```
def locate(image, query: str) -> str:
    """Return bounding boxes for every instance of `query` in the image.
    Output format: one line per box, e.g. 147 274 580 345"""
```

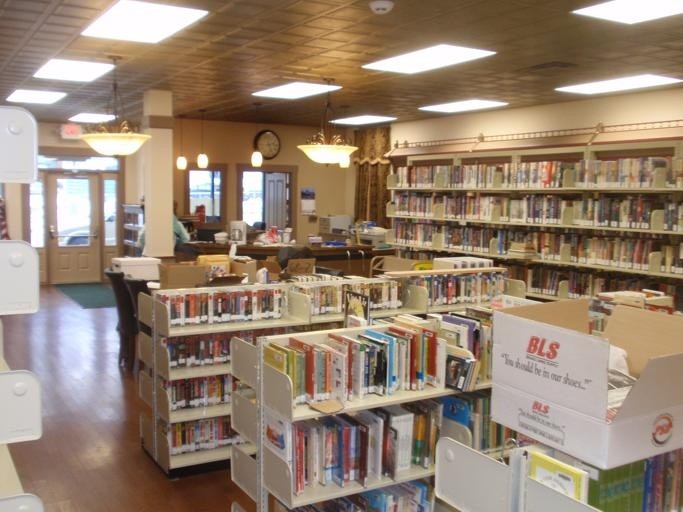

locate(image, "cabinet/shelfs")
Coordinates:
319 214 351 242
432 426 683 512
0 104 45 511
230 304 498 512
120 203 145 257
387 147 683 314
137 266 526 476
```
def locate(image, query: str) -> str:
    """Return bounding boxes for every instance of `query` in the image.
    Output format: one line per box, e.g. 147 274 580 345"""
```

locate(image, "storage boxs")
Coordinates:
490 298 683 470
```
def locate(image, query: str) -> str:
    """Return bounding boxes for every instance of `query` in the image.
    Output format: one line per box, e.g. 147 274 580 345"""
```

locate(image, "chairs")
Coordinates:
102 267 148 371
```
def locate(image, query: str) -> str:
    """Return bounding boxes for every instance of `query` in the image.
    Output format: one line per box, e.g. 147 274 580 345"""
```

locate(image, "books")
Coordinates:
160 415 250 458
291 388 517 499
396 245 441 261
395 190 500 221
393 217 683 276
405 271 507 309
262 304 494 406
395 156 499 189
494 258 682 337
163 322 343 371
288 272 407 316
501 154 683 191
159 372 242 413
502 192 683 231
525 447 683 512
156 282 290 327
291 478 437 512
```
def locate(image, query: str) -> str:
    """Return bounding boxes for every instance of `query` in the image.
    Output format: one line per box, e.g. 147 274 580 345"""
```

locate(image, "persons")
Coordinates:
137 213 190 256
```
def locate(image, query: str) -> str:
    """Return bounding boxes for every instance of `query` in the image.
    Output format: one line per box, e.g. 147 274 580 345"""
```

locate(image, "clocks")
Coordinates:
253 130 281 160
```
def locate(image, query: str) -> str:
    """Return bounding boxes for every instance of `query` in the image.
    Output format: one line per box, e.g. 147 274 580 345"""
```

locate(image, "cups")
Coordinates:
283 233 290 244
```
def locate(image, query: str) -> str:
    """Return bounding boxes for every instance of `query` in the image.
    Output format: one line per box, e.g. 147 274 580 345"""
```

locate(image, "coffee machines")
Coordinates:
229 221 246 245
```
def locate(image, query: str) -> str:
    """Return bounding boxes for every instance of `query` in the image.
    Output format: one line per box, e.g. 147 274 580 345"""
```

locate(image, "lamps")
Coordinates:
296 78 358 169
250 103 264 169
79 54 152 156
176 114 187 170
196 109 209 169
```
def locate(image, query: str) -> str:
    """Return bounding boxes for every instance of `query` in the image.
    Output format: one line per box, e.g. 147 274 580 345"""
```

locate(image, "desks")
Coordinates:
188 241 373 259
